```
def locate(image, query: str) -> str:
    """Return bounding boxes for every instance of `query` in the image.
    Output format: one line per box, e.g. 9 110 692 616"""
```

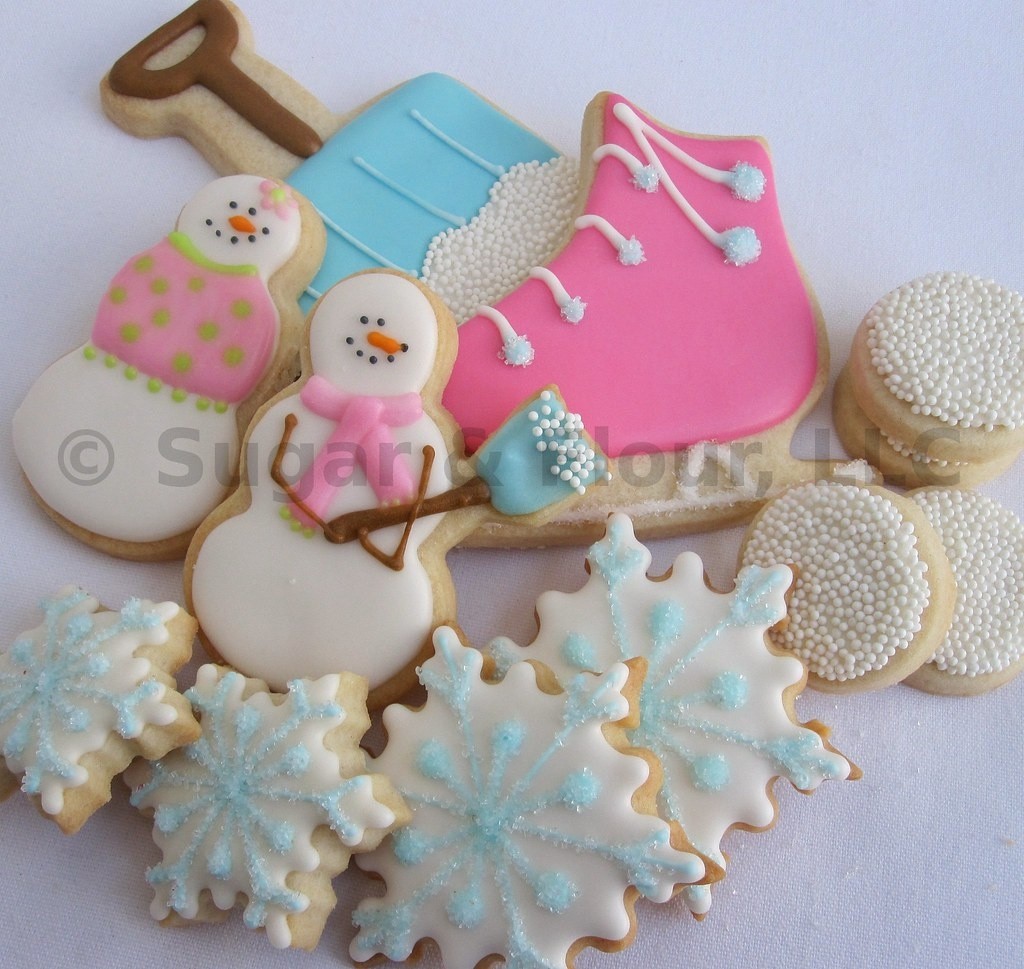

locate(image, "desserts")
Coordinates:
2 93 1022 969
